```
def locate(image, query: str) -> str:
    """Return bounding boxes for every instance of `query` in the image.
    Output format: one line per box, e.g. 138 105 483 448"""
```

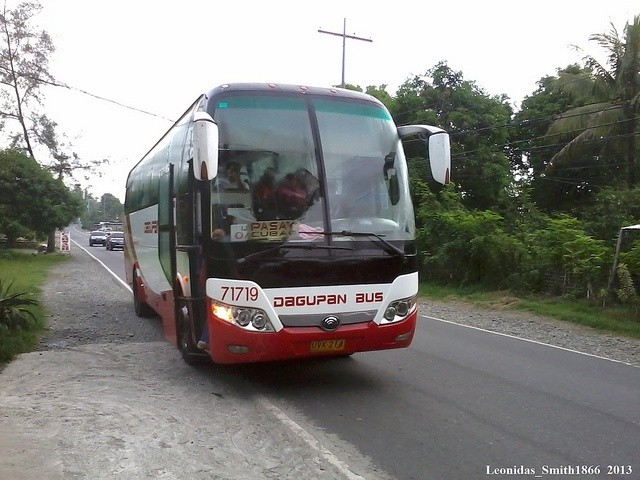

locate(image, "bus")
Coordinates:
123 81 451 365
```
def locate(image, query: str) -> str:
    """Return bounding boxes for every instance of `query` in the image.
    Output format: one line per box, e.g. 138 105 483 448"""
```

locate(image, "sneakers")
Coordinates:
198 341 209 350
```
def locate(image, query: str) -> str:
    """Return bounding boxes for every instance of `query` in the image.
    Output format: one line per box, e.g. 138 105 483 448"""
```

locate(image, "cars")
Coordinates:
106 232 124 251
89 231 107 246
96 228 112 232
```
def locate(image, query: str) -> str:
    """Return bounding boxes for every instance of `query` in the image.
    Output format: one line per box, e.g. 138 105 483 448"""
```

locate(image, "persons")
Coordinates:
214 160 247 193
343 185 378 219
213 214 243 242
